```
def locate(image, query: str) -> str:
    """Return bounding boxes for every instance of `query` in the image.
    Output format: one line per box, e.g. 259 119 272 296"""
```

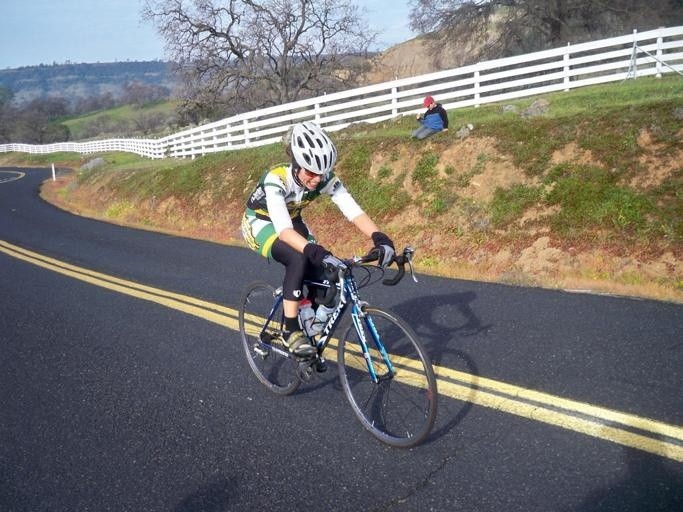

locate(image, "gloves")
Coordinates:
370 230 395 271
304 243 340 282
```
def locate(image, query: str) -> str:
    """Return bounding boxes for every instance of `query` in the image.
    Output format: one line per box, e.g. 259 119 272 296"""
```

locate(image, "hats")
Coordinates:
424 96 434 107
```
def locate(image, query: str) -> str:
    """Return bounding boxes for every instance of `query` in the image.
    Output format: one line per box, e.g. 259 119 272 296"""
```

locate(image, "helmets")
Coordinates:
289 121 338 174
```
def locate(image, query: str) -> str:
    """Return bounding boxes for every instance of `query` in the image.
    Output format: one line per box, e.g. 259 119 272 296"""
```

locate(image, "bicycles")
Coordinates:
235 246 439 450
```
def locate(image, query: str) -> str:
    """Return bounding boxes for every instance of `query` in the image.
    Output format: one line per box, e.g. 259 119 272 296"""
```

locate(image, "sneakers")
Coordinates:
314 358 327 372
280 330 317 359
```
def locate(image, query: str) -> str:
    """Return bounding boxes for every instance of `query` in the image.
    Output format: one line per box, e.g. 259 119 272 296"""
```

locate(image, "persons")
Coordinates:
238 122 396 374
408 96 449 143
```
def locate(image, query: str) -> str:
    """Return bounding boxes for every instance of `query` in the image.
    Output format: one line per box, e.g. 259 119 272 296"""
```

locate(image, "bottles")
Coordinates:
310 298 336 333
297 296 318 338
